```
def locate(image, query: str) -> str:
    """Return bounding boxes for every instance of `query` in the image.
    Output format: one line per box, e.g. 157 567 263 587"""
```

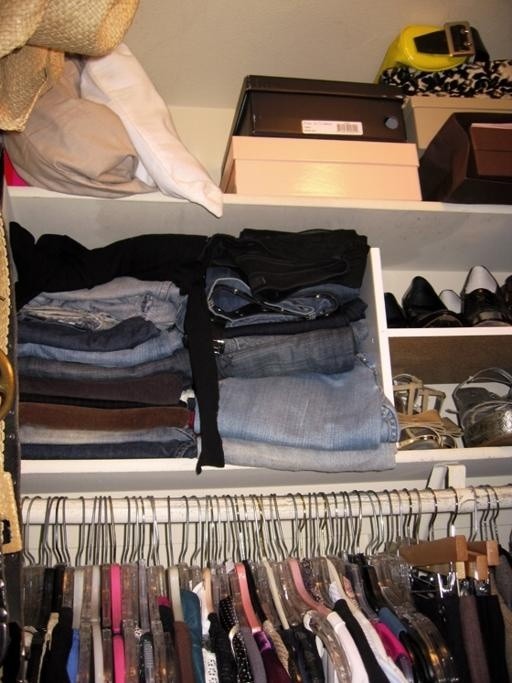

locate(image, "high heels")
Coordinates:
445 366 512 447
384 265 512 328
393 374 464 449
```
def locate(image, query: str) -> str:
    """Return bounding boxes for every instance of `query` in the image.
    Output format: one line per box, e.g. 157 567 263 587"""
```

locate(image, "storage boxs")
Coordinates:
218 75 512 205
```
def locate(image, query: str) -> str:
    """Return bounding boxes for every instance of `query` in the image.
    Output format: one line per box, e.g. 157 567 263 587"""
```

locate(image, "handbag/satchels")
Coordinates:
374 20 474 86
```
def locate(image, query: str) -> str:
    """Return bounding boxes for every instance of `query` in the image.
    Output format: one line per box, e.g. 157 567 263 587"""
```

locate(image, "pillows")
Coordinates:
78 37 225 221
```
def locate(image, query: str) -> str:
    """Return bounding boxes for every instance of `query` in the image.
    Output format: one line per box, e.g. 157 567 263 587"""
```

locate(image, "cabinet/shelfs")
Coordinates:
2 184 512 474
